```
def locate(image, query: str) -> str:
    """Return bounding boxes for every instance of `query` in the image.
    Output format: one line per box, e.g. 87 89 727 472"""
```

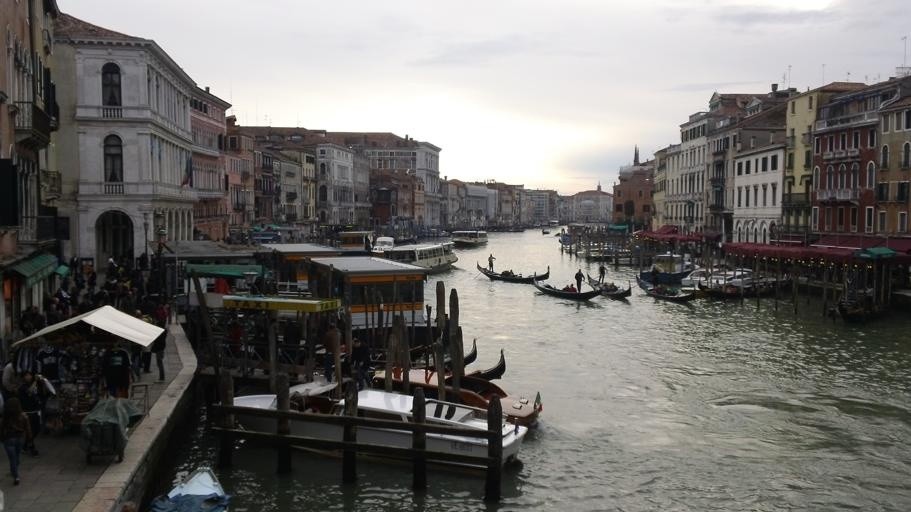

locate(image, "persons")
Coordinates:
598 263 608 283
1 221 445 486
864 283 874 313
488 254 497 273
568 284 576 291
651 266 660 292
574 269 585 293
563 285 569 291
713 279 723 289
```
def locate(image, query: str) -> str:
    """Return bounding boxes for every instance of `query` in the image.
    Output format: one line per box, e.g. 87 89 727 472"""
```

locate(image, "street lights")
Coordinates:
140 211 150 272
158 224 169 325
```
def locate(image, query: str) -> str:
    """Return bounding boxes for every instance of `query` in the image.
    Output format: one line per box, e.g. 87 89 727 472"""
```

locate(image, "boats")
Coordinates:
527 241 791 304
148 463 228 512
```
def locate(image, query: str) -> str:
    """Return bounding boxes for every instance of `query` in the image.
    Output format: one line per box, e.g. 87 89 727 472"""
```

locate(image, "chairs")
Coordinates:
25 335 151 464
